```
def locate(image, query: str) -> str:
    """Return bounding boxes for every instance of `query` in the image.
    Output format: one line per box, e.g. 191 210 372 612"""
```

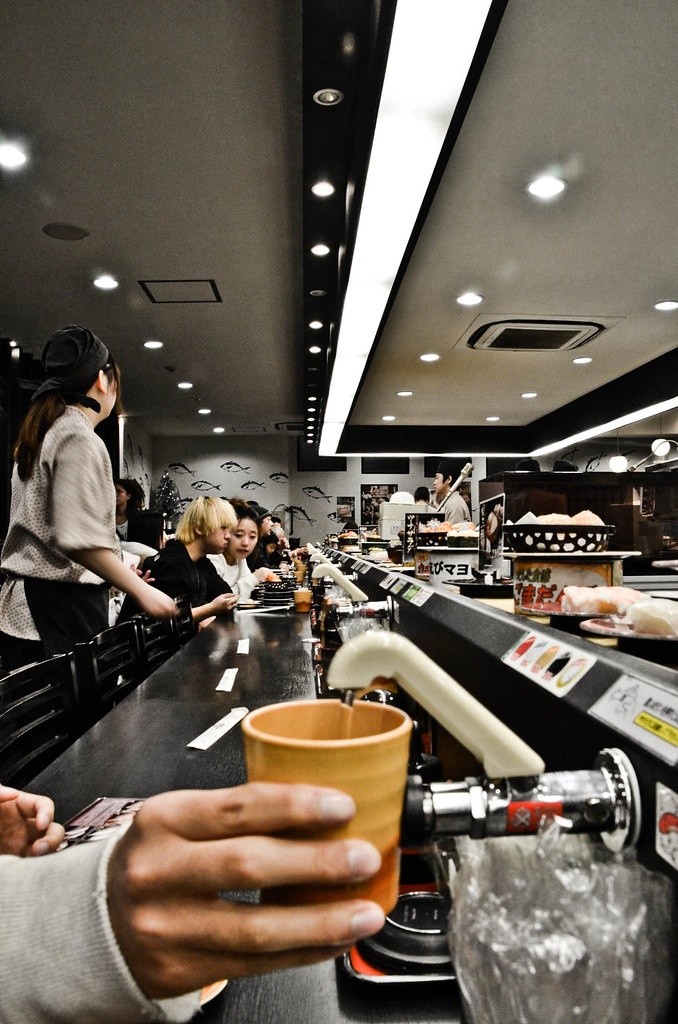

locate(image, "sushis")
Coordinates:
559 584 678 636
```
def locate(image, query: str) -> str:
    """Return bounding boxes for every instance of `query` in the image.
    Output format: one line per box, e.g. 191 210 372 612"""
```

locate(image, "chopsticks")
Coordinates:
225 594 240 604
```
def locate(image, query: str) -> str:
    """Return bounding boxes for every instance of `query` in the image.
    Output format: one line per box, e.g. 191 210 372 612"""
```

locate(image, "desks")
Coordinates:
21 607 316 825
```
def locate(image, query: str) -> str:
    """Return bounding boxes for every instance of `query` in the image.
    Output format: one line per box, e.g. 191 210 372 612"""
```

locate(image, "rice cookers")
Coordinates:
513 557 624 624
415 546 430 580
429 547 479 591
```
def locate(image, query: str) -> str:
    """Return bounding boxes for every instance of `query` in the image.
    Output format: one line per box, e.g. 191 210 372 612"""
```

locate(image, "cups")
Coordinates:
293 571 304 582
294 560 306 576
294 590 312 612
241 697 414 915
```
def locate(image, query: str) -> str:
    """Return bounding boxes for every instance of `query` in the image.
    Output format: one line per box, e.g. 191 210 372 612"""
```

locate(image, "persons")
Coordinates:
397 460 472 540
116 496 309 632
116 477 160 549
0 781 386 1024
0 325 181 665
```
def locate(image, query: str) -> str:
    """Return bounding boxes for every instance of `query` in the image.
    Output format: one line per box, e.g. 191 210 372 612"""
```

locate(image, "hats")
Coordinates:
251 505 272 520
437 460 462 479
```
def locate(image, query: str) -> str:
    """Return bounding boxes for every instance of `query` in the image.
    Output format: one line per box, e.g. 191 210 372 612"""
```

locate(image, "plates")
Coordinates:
238 603 262 608
520 602 607 637
580 617 678 662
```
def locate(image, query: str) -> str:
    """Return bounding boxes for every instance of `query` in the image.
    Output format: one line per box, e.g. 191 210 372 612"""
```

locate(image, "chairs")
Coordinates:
0 595 196 791
